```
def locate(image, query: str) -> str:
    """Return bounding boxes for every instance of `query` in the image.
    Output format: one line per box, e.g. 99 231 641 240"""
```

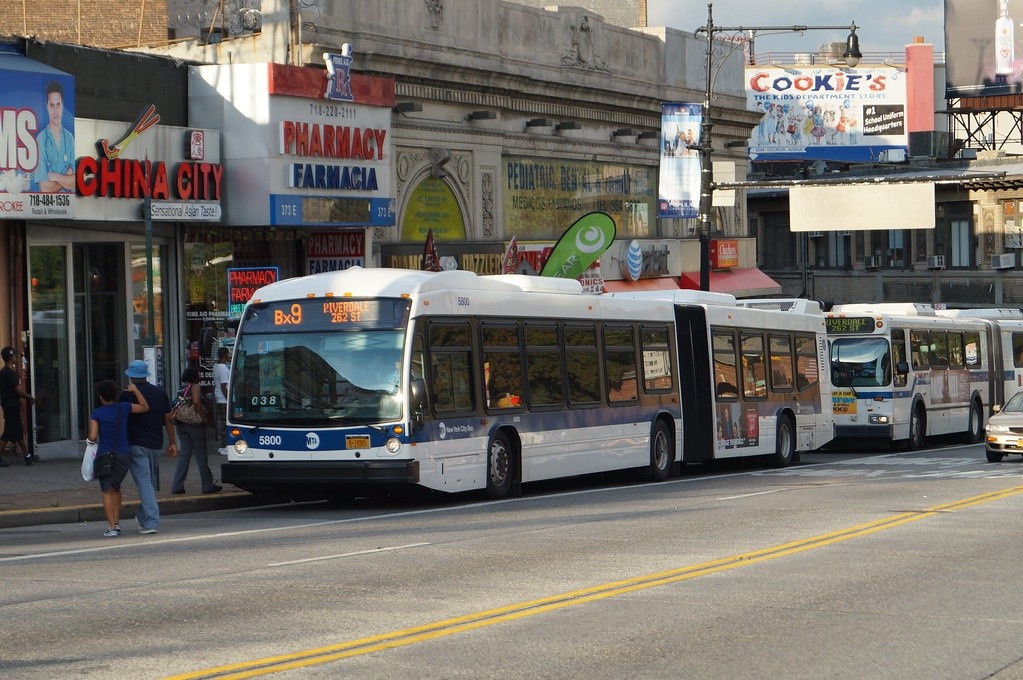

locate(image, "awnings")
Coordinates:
682 267 782 298
602 278 680 293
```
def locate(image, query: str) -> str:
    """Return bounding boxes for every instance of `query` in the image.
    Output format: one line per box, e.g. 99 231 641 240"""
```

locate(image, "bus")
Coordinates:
221 264 835 516
131 255 163 345
826 302 1023 450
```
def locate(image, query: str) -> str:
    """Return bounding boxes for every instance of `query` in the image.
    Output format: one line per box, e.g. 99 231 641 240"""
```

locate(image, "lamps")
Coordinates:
467 110 496 122
526 118 552 128
613 128 639 137
556 121 582 131
638 131 662 138
724 140 749 148
394 102 423 115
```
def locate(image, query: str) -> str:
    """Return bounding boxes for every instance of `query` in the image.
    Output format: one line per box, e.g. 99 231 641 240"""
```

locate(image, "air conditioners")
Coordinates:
808 231 824 237
837 231 852 236
926 255 945 267
991 253 1015 269
864 256 882 267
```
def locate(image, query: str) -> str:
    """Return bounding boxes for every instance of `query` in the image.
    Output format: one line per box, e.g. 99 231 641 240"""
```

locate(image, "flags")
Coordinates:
503 238 520 274
421 228 440 271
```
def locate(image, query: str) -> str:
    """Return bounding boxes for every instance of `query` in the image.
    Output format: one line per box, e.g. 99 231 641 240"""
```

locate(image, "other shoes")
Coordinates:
138 527 158 534
217 448 227 455
25 454 39 464
171 489 186 494
201 484 222 493
0 458 9 467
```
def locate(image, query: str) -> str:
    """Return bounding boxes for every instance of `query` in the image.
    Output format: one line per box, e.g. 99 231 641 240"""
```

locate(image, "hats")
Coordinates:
124 360 150 378
1 347 18 361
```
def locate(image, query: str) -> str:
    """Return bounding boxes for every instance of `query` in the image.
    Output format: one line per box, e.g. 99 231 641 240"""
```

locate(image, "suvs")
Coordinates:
33 309 67 338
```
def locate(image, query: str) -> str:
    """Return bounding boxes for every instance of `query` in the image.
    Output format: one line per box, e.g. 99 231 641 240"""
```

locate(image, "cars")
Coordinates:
983 386 1023 462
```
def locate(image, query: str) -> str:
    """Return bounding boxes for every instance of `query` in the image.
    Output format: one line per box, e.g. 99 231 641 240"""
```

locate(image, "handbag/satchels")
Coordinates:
92 452 114 480
170 384 202 425
80 439 98 481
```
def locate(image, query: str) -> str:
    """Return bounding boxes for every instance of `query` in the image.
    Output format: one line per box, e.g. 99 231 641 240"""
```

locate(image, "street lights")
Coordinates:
697 23 863 289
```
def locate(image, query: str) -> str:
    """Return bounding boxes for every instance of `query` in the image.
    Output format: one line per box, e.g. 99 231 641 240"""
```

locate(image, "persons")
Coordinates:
171 368 223 494
841 363 871 376
212 347 230 455
88 380 149 536
717 407 748 450
664 125 698 156
118 360 177 534
0 346 39 467
34 80 75 193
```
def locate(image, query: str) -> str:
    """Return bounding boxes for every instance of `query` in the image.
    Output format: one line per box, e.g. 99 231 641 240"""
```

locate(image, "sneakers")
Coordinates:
103 530 117 537
108 524 120 533
